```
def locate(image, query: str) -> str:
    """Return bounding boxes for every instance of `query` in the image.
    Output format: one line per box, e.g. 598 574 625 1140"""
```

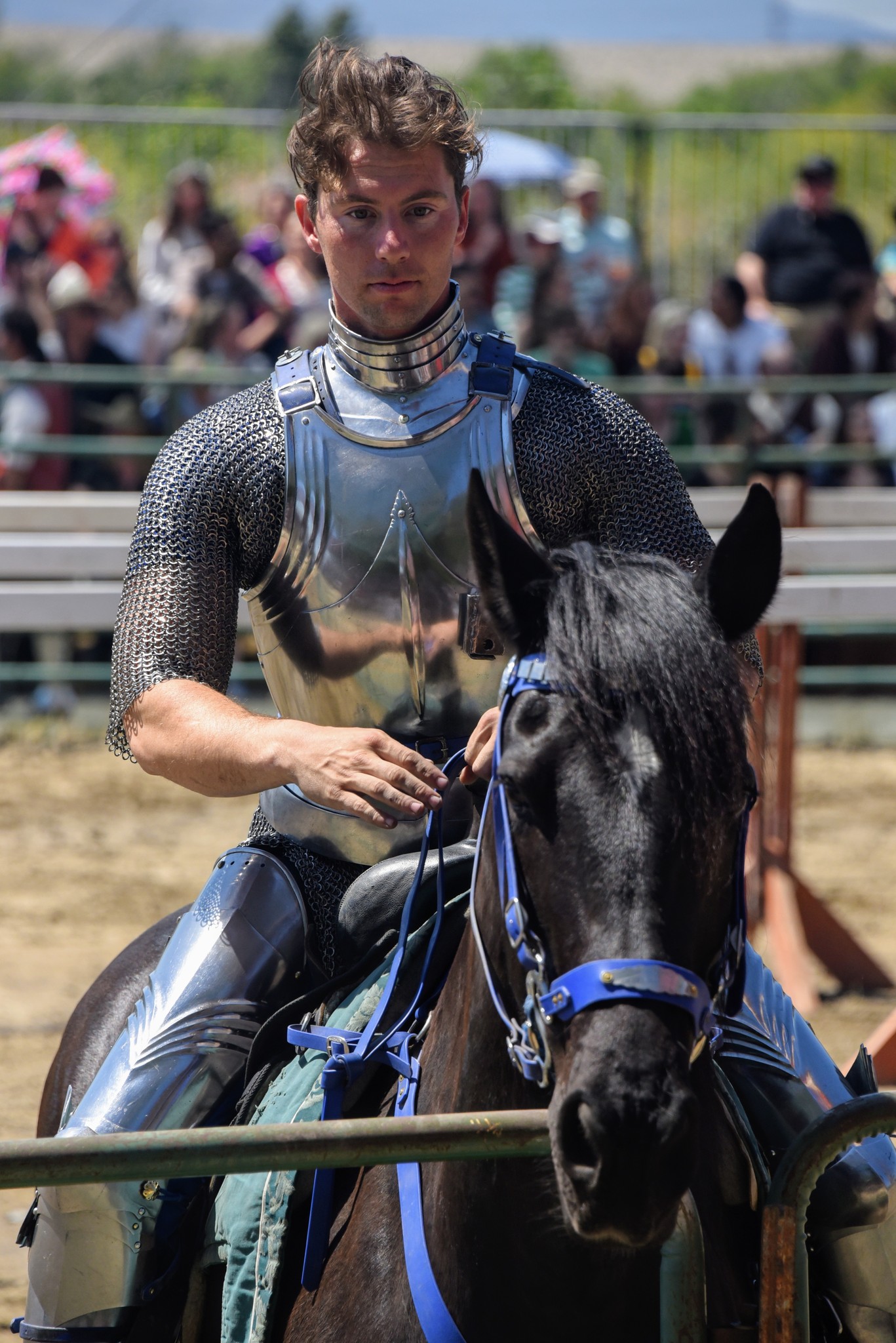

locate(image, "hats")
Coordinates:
560 156 603 199
797 158 836 185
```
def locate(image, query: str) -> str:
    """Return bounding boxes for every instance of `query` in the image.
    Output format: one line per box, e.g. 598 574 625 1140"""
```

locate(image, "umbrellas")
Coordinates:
465 129 578 182
0 123 115 219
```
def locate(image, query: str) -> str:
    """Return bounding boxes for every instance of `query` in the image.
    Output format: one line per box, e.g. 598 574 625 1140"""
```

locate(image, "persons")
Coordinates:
12 35 896 1343
0 155 896 528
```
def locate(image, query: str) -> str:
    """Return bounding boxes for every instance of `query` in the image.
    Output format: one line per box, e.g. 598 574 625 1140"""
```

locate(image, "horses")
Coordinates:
33 466 784 1343
